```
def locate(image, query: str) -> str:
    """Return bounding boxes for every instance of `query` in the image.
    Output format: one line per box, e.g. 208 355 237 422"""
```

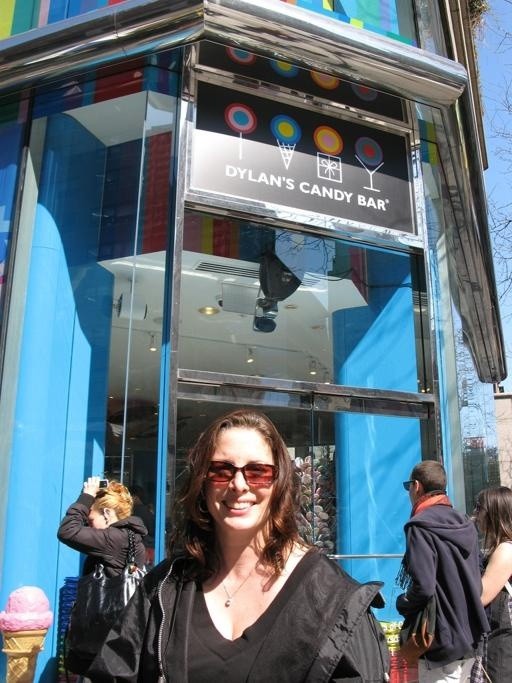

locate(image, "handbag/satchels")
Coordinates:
71 565 145 654
399 594 436 664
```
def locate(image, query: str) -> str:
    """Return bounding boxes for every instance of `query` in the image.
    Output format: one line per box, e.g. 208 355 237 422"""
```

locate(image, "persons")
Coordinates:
393 460 490 682
78 406 393 683
470 489 510 682
58 476 149 682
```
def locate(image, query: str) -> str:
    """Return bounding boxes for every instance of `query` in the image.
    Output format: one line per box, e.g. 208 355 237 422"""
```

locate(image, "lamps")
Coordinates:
246 345 256 363
148 331 158 352
309 356 317 375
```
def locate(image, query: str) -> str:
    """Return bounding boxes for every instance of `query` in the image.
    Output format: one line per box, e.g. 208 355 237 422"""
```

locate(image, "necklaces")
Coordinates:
217 561 263 606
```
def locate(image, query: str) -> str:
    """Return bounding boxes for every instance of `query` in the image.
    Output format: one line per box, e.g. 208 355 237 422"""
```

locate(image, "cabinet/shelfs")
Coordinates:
105 451 135 492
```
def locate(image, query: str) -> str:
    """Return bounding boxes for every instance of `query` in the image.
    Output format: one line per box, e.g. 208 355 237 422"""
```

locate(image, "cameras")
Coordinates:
100 480 108 489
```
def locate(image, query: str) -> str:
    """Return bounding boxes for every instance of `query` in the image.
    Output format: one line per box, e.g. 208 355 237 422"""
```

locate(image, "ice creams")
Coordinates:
0 586 53 683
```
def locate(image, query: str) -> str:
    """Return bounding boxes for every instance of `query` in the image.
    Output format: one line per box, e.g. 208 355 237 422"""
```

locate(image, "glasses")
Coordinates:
207 461 277 483
403 480 414 490
